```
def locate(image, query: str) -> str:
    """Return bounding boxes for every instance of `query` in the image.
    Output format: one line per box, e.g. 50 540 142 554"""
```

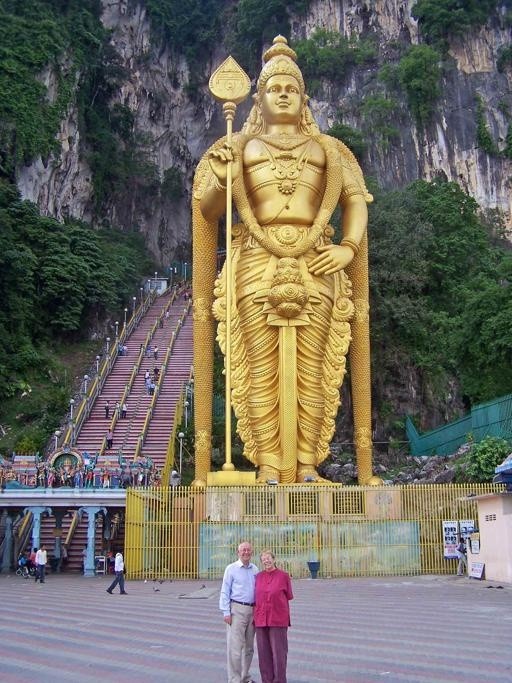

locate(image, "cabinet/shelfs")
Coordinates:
95 556 107 576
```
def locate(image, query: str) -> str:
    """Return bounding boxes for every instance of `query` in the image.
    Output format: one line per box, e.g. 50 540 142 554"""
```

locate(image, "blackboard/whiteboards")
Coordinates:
469 562 484 579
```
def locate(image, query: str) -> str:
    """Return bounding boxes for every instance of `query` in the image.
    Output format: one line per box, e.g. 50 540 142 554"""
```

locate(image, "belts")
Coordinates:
229 597 256 608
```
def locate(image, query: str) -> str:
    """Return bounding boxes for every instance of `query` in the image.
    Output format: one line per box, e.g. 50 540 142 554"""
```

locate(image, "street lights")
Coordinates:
189 378 194 413
176 430 185 475
115 262 188 337
105 336 111 353
84 373 89 394
54 430 61 450
184 400 190 429
96 355 101 373
69 397 75 420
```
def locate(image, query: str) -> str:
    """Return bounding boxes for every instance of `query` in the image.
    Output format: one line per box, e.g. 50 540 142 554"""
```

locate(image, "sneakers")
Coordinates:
106 590 113 594
120 591 127 594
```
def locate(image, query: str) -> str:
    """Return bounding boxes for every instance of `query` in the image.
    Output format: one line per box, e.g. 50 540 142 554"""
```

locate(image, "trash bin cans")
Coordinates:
48 558 60 572
307 562 320 579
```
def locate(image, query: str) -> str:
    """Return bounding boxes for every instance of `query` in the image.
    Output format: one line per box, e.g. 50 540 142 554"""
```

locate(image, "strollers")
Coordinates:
16 554 36 576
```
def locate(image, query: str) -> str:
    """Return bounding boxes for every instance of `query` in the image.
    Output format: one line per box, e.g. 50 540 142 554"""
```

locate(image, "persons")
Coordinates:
254 549 293 683
456 537 468 576
219 542 260 683
200 34 367 487
